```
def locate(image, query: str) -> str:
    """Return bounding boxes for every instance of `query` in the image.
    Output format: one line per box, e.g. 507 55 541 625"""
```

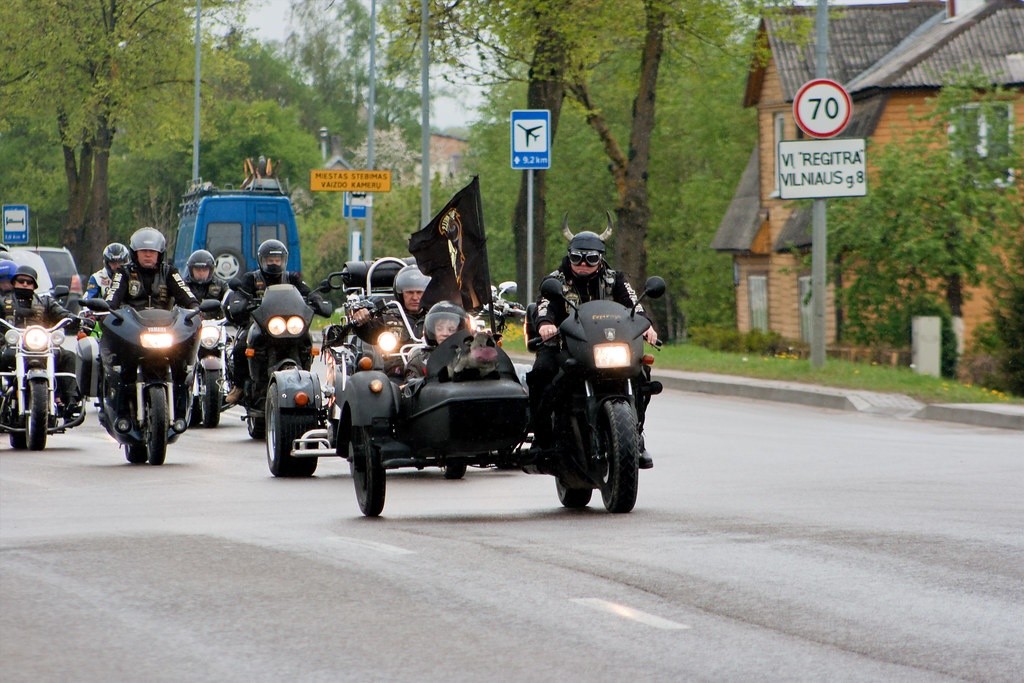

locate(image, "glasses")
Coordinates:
569 248 601 266
15 277 34 284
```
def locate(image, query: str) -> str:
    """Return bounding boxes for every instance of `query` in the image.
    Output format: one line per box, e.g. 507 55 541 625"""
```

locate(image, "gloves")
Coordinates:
66 313 80 330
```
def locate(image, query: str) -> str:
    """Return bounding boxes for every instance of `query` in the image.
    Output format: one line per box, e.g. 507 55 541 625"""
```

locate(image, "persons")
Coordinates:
0 227 474 420
521 230 657 469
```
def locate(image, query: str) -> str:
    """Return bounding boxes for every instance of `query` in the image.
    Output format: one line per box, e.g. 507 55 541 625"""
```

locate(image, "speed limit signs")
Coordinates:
791 78 855 139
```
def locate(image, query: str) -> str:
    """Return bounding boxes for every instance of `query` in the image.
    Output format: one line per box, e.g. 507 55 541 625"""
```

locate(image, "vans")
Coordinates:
172 178 302 282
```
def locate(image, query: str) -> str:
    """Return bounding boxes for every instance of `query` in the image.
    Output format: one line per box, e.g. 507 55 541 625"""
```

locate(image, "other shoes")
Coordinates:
225 385 244 403
57 403 84 424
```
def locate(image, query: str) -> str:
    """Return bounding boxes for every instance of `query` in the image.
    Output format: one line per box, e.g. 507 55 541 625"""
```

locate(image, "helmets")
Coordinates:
102 243 130 279
567 230 606 283
10 265 38 289
392 265 432 304
423 300 468 345
257 239 289 279
129 227 166 275
0 260 18 282
0 250 13 261
187 249 217 288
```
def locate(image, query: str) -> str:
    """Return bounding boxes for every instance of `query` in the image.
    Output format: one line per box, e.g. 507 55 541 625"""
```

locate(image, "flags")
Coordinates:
407 175 492 319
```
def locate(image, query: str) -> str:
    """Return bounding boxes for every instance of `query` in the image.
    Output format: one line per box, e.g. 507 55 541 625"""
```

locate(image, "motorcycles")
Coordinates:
0 311 86 450
86 297 220 465
264 257 669 517
227 272 346 439
65 299 240 428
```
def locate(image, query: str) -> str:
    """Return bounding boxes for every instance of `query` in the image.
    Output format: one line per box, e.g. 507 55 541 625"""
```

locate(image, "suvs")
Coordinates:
13 246 84 311
